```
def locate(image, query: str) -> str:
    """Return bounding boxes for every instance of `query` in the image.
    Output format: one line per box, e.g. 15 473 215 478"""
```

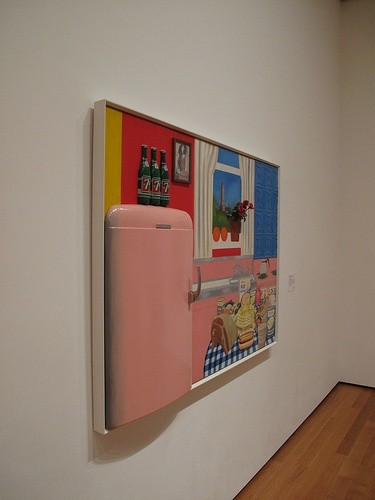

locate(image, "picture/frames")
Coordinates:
91 98 282 434
